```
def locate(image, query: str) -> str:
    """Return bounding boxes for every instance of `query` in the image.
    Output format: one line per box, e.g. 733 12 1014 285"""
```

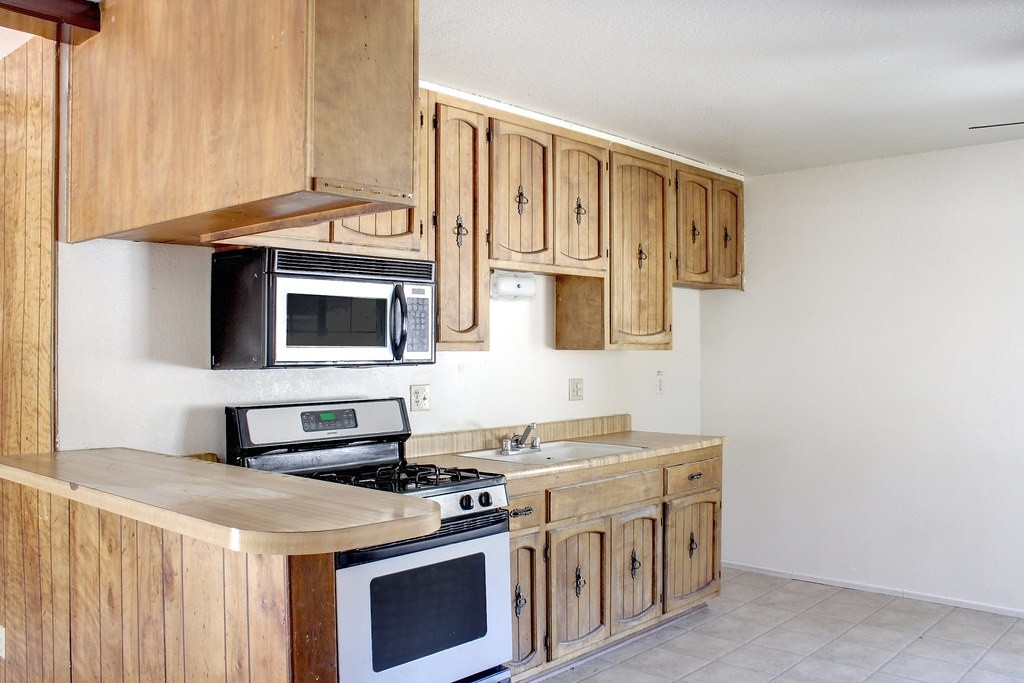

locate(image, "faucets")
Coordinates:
500 422 542 455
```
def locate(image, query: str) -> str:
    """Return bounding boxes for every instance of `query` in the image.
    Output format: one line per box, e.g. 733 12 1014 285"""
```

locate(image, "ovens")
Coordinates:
334 510 512 683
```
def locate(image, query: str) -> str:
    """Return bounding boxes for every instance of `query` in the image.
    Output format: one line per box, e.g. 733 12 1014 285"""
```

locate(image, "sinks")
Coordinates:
458 438 646 465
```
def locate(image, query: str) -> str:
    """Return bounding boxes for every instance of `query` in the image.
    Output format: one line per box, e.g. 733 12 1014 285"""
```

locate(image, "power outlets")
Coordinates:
569 377 583 401
410 383 431 411
657 370 664 395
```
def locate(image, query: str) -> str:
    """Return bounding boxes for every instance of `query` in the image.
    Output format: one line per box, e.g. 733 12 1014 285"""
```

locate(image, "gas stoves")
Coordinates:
224 398 510 520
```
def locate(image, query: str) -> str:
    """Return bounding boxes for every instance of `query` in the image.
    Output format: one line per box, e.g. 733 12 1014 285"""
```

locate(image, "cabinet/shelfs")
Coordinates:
503 474 550 683
66 0 422 246
546 455 666 666
660 444 723 619
210 78 425 261
489 106 611 278
425 88 489 351
670 158 744 292
553 124 675 351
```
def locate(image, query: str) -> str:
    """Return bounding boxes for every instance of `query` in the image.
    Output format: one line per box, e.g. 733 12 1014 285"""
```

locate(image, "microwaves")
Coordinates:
210 247 438 370
